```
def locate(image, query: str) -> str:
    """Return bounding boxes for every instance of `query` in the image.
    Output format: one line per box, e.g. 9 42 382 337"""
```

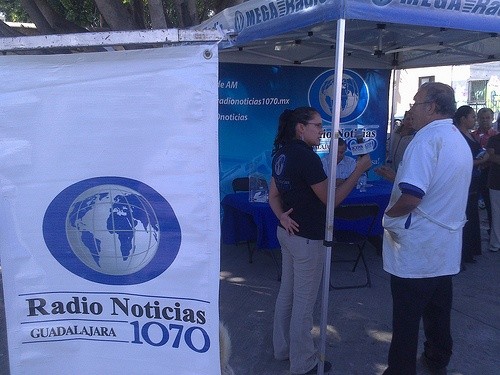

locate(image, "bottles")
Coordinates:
356 173 367 192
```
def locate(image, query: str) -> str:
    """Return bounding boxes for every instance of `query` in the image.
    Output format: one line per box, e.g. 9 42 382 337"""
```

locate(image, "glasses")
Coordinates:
301 121 323 128
409 102 435 110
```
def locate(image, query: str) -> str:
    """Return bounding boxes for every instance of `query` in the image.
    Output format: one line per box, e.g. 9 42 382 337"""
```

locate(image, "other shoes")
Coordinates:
489 245 499 252
465 254 477 263
304 360 332 375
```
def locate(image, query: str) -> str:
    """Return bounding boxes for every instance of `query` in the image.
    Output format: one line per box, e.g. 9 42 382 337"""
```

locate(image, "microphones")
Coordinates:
351 129 374 157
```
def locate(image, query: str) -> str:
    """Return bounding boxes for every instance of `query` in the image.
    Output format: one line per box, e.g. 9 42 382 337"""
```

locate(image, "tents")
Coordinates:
191 0 500 375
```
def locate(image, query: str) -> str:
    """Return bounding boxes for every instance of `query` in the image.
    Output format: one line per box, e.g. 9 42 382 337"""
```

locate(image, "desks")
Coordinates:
221 180 393 282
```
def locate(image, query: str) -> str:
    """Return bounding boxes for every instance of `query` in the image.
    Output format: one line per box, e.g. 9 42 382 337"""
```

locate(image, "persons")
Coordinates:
321 137 357 187
392 111 416 172
454 106 500 270
374 82 473 375
269 107 372 375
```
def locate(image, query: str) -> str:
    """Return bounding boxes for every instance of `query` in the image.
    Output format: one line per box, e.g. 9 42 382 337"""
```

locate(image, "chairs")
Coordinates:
329 203 379 289
232 177 269 264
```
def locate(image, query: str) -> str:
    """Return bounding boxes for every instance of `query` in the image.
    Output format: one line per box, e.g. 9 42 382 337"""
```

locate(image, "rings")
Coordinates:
368 163 371 166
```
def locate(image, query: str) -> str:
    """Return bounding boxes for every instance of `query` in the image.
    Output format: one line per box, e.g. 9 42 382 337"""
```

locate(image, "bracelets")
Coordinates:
354 172 360 178
